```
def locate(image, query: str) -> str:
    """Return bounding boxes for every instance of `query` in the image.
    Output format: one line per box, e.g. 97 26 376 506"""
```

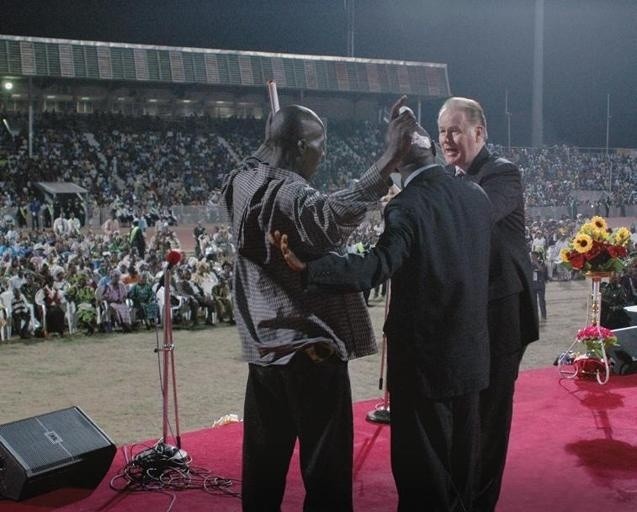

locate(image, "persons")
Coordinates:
216 94 415 511
2 94 636 340
265 120 493 511
436 96 539 512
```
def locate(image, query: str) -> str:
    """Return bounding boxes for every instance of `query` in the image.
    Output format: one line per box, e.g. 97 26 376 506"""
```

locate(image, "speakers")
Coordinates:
0 406 118 502
624 305 637 327
602 327 637 377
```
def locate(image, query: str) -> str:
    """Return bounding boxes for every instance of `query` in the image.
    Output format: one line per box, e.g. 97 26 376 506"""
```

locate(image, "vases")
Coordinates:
574 357 612 379
588 277 603 328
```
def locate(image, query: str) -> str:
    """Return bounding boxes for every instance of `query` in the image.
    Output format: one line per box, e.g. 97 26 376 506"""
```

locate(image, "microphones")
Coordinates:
265 78 282 115
157 244 183 294
390 183 401 195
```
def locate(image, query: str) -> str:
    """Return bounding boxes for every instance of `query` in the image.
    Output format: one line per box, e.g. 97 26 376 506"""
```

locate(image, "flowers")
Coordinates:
577 321 621 360
560 217 633 281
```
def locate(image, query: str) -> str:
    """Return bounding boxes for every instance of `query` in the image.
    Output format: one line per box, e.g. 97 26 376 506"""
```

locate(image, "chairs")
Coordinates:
0 249 234 342
